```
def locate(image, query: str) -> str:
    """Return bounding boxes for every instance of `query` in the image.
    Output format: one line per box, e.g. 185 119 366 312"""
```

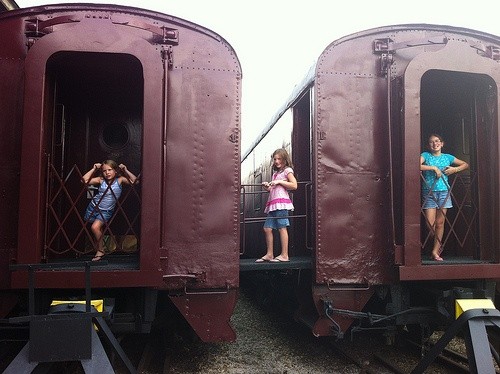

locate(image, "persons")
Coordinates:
254 148 298 262
80 160 138 263
419 133 469 260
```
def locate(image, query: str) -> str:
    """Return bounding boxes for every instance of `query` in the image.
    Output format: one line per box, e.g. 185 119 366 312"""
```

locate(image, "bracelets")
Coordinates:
454 167 461 173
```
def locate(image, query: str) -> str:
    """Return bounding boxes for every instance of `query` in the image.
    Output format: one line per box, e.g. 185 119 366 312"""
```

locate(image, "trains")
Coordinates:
0 2 243 374
241 23 500 374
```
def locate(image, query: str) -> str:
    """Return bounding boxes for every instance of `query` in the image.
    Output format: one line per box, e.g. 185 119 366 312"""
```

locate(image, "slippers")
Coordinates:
270 257 290 263
429 254 443 261
255 258 274 263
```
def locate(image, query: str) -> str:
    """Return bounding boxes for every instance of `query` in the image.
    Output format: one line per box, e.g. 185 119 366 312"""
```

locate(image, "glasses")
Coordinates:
430 139 441 143
